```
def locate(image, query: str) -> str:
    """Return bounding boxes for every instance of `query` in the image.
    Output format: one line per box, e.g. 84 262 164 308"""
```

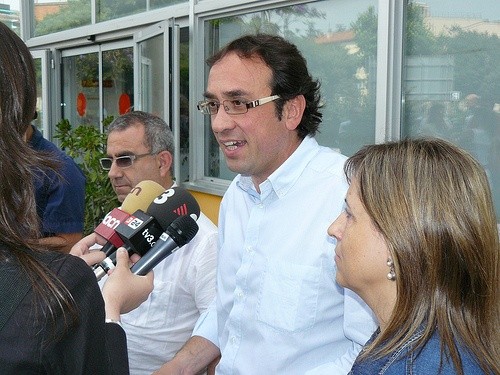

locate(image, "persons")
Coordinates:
21 114 86 250
196 33 381 375
0 18 155 375
327 137 500 375
80 111 221 375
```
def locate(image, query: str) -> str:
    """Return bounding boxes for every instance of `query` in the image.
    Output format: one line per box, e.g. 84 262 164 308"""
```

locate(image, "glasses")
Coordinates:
197 95 281 116
98 153 155 171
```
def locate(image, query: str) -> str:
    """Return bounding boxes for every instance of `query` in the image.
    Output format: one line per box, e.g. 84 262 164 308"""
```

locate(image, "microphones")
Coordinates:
129 215 198 275
93 186 200 282
89 180 166 271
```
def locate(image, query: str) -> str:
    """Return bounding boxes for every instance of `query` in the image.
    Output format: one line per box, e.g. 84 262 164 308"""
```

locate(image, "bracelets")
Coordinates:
105 318 123 329
